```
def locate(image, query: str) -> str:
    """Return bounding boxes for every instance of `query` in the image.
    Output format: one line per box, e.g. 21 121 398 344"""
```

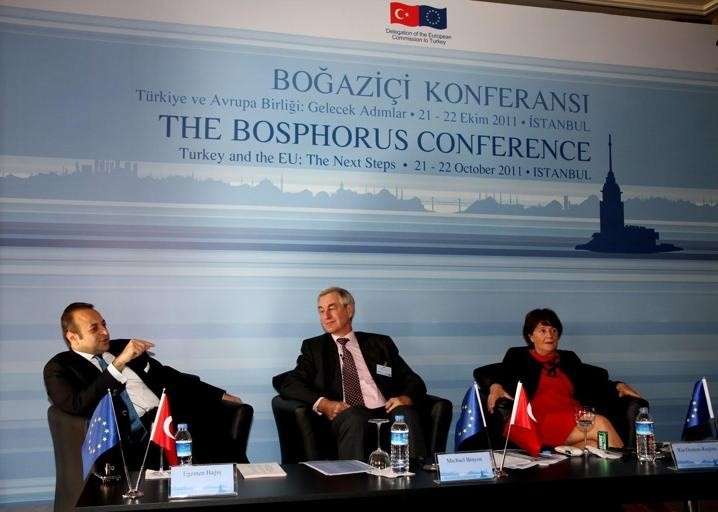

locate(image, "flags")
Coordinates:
150 393 179 466
81 394 119 482
501 381 542 458
681 377 716 441
454 383 487 451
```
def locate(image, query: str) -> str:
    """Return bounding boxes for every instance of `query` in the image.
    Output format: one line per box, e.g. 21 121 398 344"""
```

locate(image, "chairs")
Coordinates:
269 394 454 468
468 389 649 455
47 397 254 512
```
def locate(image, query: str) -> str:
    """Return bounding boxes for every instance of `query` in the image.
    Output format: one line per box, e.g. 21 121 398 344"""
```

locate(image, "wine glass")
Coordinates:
367 418 390 469
575 405 596 452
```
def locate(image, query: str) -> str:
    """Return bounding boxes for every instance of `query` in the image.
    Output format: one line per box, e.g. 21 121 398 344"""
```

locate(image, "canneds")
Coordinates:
597 431 607 450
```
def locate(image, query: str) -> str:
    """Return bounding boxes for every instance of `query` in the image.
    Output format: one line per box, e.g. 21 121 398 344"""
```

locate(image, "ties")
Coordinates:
337 336 365 409
92 353 148 443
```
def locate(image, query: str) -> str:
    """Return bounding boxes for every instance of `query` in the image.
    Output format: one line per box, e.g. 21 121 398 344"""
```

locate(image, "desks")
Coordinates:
82 440 718 512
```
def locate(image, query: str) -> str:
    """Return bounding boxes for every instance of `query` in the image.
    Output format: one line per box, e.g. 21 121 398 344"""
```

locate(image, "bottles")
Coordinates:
390 414 410 474
634 406 657 463
172 422 193 467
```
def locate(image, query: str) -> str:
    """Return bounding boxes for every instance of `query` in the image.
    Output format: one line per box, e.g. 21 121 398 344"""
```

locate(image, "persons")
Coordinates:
484 306 646 450
40 302 251 470
271 286 434 461
